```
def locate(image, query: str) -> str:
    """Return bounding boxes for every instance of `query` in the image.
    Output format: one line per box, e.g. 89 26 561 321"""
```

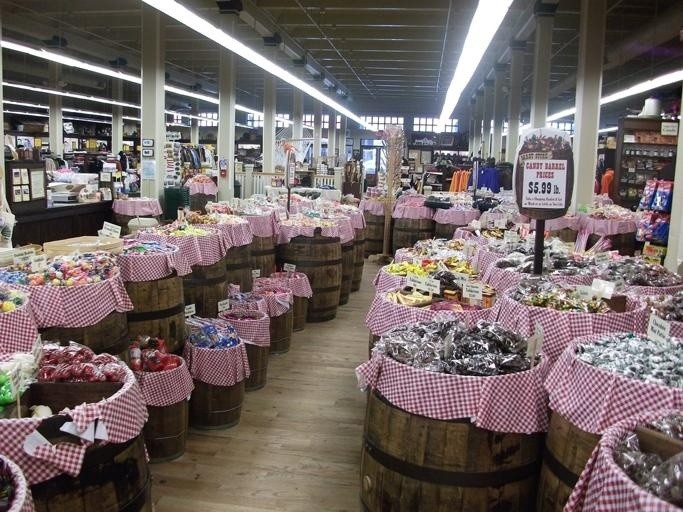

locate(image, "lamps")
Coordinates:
439 0 514 126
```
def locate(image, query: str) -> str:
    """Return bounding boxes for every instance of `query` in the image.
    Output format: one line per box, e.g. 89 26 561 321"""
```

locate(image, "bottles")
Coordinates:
16 145 42 161
625 145 673 158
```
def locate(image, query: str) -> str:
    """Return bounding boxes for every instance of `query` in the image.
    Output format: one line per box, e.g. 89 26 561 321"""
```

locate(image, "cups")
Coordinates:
18 125 24 133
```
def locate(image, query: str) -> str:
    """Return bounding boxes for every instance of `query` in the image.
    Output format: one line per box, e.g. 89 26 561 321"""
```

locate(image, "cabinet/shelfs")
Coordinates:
613 118 680 207
5 160 47 216
